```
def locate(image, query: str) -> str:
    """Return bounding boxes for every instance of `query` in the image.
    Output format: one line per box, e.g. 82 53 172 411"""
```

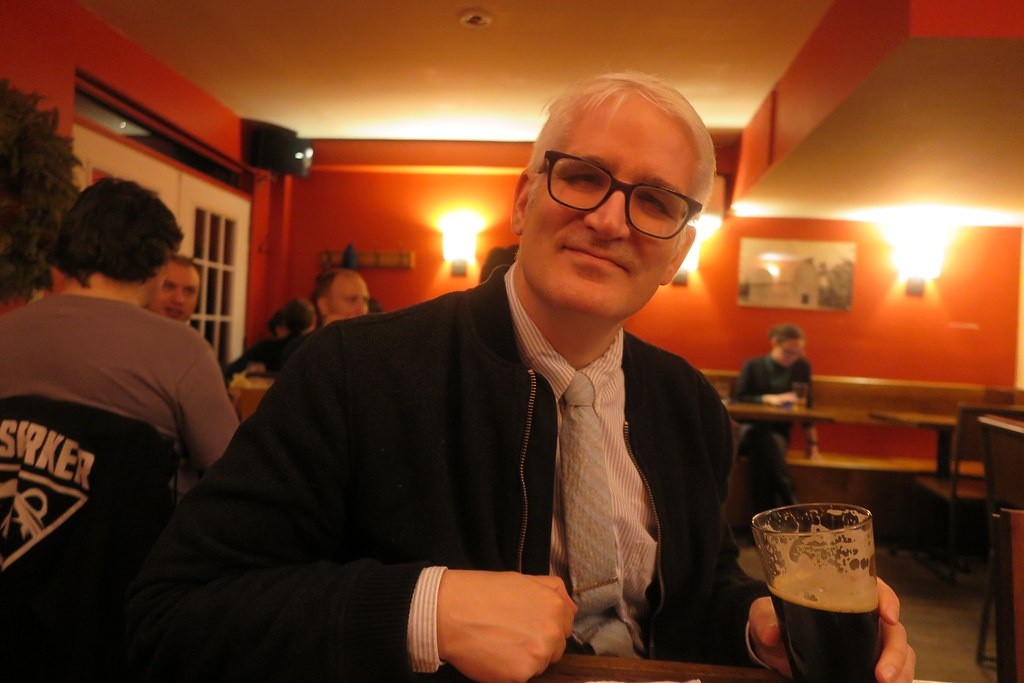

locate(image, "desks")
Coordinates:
722 402 843 424
868 409 974 576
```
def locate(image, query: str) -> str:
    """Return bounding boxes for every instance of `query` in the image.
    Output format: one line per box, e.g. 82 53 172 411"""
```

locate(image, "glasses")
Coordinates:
538 150 702 239
779 344 805 360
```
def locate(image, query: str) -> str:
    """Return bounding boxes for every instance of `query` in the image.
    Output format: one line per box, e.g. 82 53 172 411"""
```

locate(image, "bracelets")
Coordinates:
806 440 815 445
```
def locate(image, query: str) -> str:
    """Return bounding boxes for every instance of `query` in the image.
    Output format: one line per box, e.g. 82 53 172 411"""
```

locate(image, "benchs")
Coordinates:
698 372 984 547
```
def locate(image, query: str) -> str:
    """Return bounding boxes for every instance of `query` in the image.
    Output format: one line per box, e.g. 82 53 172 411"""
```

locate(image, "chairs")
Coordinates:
914 404 1024 683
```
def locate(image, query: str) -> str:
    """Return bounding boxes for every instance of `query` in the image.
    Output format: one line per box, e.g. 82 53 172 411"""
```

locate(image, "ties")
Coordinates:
560 371 642 660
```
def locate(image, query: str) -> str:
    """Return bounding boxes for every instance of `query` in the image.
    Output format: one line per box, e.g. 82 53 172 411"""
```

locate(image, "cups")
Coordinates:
231 378 275 420
793 383 806 408
751 503 879 683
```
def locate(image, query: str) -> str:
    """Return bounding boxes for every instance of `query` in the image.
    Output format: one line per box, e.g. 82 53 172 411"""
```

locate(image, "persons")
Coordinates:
728 323 819 545
119 70 916 683
0 77 241 683
